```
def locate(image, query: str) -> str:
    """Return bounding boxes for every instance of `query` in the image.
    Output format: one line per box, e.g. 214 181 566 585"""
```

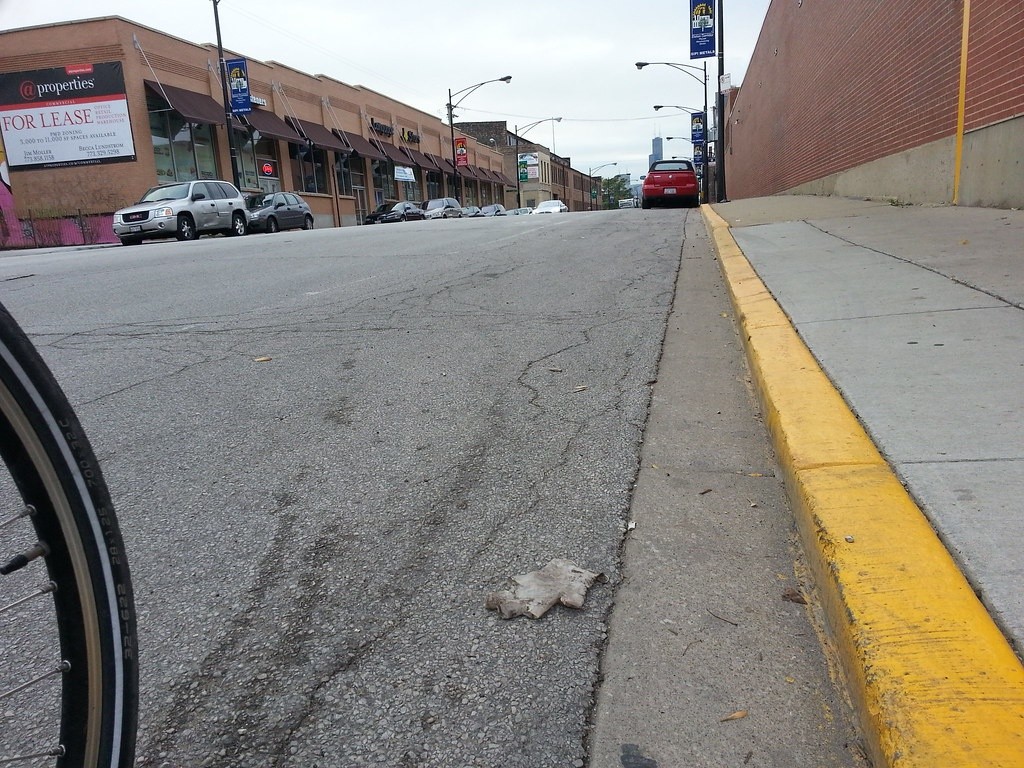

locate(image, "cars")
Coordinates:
111 179 251 245
365 201 426 224
514 207 533 215
248 191 314 234
420 198 463 219
481 204 507 216
639 159 704 209
535 200 568 214
461 206 484 217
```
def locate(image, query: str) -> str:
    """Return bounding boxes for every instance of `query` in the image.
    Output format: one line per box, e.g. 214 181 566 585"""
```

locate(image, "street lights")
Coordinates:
608 173 631 210
448 76 512 203
588 163 618 211
515 117 563 209
635 60 709 204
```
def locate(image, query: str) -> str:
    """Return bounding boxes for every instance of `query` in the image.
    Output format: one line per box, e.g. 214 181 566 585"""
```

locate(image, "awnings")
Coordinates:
400 146 440 174
446 157 478 181
480 167 505 185
333 127 388 162
143 78 247 131
424 153 461 178
285 115 351 155
466 164 490 182
241 107 306 146
370 138 416 169
493 170 516 188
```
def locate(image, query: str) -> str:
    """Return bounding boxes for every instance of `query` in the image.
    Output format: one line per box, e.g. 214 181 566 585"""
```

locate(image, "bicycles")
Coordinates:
1 302 140 768
